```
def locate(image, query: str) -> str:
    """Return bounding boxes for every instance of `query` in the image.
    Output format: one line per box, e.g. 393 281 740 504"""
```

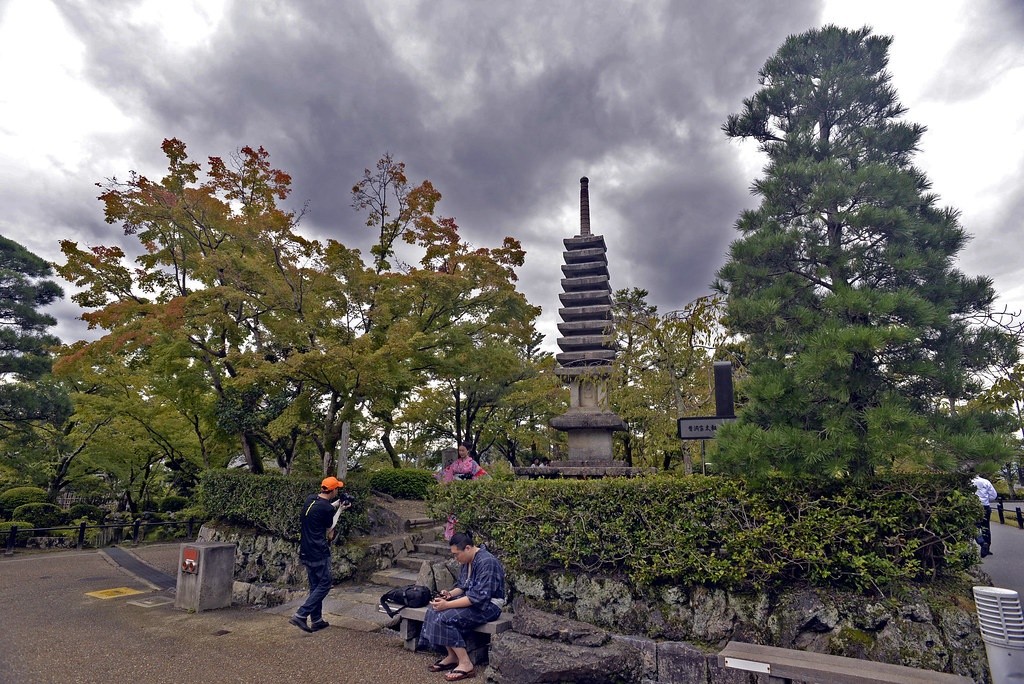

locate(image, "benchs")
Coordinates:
376 599 509 653
719 641 976 684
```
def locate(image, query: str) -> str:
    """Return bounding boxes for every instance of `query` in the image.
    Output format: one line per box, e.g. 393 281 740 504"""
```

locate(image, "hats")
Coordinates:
321 477 344 491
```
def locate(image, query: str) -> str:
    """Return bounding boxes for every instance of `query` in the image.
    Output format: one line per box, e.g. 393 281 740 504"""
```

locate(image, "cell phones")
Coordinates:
439 594 447 598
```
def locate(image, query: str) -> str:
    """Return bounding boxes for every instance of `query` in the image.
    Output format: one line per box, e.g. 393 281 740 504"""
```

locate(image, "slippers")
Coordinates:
428 659 457 672
445 667 476 681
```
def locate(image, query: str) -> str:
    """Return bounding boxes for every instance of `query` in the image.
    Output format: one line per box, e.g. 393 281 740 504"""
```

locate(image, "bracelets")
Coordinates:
448 592 452 598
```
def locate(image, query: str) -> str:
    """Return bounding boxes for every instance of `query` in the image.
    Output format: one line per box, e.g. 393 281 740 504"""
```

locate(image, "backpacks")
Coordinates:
380 583 431 618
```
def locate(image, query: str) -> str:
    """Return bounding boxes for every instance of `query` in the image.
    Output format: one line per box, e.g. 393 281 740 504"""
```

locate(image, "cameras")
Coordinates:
339 492 355 506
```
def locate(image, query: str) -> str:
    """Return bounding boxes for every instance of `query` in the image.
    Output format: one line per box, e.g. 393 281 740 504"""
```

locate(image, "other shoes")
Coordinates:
311 620 329 631
988 551 992 555
289 615 313 633
981 543 988 557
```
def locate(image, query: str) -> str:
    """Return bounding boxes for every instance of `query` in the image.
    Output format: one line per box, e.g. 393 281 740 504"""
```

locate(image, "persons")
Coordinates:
969 467 998 558
531 456 553 467
289 476 352 634
437 443 493 543
417 532 505 682
